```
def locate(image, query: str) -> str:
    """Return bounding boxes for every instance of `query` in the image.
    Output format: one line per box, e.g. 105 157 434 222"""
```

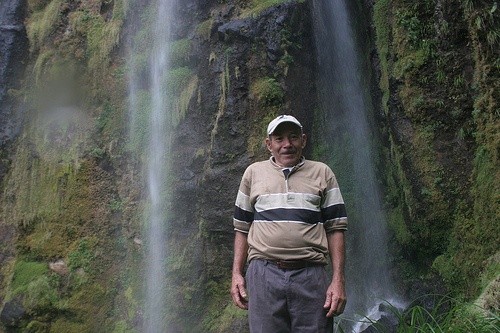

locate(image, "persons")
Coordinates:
229 113 349 333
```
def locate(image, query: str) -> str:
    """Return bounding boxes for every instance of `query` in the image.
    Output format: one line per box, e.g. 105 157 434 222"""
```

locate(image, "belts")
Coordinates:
265 257 326 271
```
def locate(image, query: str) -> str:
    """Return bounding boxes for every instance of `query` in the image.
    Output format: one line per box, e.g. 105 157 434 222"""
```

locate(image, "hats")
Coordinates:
266 114 303 141
